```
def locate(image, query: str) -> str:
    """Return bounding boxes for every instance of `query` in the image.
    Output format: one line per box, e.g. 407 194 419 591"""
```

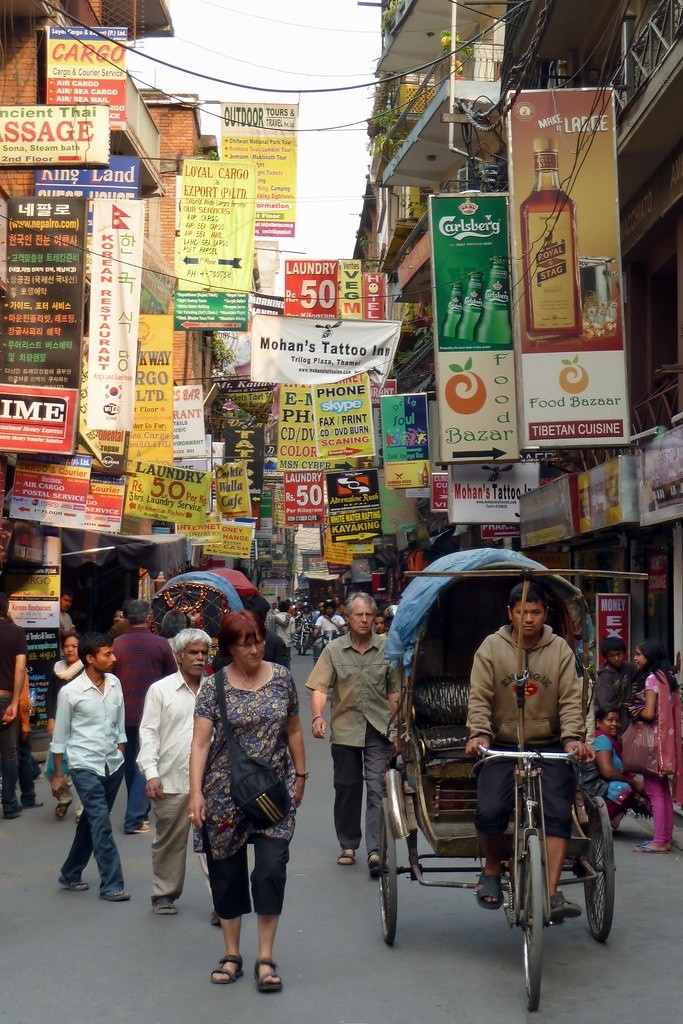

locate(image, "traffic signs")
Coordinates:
435 352 521 463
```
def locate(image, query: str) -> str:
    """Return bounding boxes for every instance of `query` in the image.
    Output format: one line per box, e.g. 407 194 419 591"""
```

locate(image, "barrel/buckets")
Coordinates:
578 255 615 308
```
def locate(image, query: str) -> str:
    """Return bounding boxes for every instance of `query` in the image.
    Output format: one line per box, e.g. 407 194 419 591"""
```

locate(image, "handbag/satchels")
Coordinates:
44 751 69 776
620 720 659 775
227 739 290 829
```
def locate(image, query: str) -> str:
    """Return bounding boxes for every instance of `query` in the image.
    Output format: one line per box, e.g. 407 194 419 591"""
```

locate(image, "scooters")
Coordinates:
315 629 344 655
295 617 315 655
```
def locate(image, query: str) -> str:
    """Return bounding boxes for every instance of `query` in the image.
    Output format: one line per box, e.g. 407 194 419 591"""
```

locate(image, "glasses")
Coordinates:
234 640 266 650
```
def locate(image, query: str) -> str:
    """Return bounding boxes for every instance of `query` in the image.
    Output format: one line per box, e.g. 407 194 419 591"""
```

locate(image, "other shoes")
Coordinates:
126 820 151 833
5 806 23 819
23 801 44 807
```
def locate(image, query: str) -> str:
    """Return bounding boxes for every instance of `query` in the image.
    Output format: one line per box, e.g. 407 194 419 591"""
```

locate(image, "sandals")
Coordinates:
211 955 243 984
367 851 381 877
255 960 282 990
338 847 356 865
100 891 131 900
57 879 89 890
56 801 72 818
75 816 80 823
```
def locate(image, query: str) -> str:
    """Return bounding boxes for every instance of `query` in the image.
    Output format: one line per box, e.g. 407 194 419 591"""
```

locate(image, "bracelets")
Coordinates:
312 716 321 723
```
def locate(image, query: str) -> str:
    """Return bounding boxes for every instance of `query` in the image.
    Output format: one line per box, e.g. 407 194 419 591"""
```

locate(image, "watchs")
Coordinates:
296 772 308 779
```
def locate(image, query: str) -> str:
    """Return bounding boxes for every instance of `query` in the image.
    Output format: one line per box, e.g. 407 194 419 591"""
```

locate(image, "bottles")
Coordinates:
442 282 463 338
473 255 513 343
455 271 483 340
520 138 583 340
29 689 37 716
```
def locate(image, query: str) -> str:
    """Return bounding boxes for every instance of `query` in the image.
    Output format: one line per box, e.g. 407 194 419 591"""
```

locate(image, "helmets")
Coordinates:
384 604 399 618
324 599 336 608
303 602 309 607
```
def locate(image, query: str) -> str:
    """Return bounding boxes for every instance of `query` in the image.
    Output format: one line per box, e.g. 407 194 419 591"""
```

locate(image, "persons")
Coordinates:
0 585 682 915
188 610 305 992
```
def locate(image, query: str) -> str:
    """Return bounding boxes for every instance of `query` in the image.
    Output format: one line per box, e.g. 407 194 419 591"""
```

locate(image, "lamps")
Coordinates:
427 155 436 161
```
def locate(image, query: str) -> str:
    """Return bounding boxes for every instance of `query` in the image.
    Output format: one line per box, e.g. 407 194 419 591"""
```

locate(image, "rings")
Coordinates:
188 814 194 819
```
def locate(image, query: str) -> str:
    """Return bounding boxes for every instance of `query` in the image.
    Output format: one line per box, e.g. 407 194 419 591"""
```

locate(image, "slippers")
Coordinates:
551 892 582 919
211 912 221 926
152 900 177 914
636 840 672 853
478 870 502 909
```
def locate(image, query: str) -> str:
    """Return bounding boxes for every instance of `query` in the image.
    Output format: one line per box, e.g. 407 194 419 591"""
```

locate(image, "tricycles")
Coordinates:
375 550 617 1013
150 569 245 679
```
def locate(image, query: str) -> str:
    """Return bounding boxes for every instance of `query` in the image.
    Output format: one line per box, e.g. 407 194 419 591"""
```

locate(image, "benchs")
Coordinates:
412 675 471 759
